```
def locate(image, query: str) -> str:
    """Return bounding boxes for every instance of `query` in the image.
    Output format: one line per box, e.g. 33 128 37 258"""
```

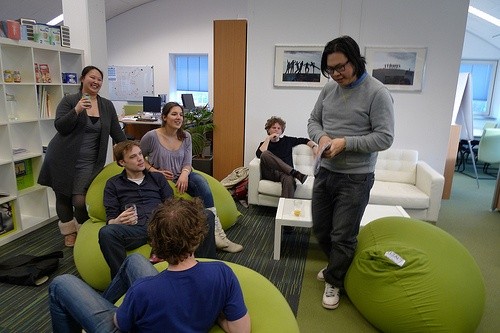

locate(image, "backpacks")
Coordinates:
220 167 248 208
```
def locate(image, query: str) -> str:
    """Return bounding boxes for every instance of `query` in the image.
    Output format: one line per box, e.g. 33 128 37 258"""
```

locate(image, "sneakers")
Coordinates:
316 262 328 281
322 282 341 309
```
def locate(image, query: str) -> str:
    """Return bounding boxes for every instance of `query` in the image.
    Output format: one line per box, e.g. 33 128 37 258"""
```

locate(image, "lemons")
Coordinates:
294 210 301 216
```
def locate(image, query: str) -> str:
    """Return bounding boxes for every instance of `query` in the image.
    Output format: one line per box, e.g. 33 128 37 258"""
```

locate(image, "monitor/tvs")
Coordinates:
142 94 167 113
182 94 195 108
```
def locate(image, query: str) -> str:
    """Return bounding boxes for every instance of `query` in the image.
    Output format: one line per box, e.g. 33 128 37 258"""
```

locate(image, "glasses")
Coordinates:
326 59 350 74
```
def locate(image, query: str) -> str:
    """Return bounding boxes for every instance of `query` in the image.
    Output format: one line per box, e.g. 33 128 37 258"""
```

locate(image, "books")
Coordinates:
37 85 51 118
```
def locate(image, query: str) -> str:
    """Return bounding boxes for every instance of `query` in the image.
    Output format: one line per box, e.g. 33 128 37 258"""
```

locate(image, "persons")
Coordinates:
307 34 395 310
97 140 217 280
310 62 320 74
255 116 319 233
285 59 290 74
298 61 303 73
48 195 252 333
295 61 300 73
141 102 243 253
36 66 130 247
290 60 296 73
304 62 310 73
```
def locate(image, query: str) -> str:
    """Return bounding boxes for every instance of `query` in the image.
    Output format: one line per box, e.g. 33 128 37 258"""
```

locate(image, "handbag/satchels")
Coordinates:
0 250 65 287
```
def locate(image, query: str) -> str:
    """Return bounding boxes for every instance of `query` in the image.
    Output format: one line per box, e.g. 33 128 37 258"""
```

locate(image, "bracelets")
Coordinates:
182 169 190 174
147 166 152 172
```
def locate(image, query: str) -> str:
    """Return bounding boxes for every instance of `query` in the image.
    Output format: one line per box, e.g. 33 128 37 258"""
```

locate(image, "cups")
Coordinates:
163 169 172 180
294 200 303 217
125 204 137 225
81 94 92 109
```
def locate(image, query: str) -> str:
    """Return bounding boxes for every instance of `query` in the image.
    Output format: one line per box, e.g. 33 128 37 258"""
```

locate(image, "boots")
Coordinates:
58 217 81 247
204 206 244 253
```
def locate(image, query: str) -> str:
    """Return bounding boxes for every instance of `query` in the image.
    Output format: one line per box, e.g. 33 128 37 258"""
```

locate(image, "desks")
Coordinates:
117 120 210 144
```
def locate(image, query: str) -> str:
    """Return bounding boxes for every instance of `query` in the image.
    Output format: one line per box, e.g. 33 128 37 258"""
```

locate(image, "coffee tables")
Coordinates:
272 198 410 260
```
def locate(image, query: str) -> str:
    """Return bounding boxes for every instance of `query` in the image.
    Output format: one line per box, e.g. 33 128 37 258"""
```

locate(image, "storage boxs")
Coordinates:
62 72 78 84
49 28 61 46
32 25 50 45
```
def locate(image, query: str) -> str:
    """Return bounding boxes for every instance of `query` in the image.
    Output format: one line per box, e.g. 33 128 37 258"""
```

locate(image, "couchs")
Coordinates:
247 145 445 228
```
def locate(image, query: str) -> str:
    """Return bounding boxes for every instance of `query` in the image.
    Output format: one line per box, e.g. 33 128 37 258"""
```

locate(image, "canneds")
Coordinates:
82 93 92 107
4 70 13 82
14 71 21 82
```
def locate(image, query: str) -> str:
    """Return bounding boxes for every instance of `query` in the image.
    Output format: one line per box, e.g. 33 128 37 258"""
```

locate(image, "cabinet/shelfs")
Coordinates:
0 38 87 247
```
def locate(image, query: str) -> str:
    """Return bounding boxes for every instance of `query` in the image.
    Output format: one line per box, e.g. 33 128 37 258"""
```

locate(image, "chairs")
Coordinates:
463 127 500 180
121 105 143 115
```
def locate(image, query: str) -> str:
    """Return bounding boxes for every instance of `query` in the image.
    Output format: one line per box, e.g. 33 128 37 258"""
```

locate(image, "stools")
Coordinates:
72 156 301 333
343 215 485 333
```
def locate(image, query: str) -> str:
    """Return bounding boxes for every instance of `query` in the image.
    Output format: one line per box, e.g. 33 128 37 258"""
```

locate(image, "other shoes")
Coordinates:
291 169 308 184
284 225 296 233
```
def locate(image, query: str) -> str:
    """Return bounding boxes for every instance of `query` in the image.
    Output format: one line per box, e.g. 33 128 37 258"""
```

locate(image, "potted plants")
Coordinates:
185 103 213 177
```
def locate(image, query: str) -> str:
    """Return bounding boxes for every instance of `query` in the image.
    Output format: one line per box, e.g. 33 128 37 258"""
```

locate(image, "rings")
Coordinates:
181 185 183 186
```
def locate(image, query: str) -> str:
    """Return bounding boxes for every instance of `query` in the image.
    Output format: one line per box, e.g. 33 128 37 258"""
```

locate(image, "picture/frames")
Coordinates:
273 45 329 87
362 44 428 93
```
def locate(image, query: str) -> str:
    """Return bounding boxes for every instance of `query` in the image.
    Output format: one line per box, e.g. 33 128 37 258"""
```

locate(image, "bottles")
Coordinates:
4 70 13 83
5 95 17 119
14 71 21 82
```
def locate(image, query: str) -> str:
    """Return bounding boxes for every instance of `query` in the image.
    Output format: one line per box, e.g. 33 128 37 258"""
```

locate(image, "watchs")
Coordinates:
312 144 318 149
